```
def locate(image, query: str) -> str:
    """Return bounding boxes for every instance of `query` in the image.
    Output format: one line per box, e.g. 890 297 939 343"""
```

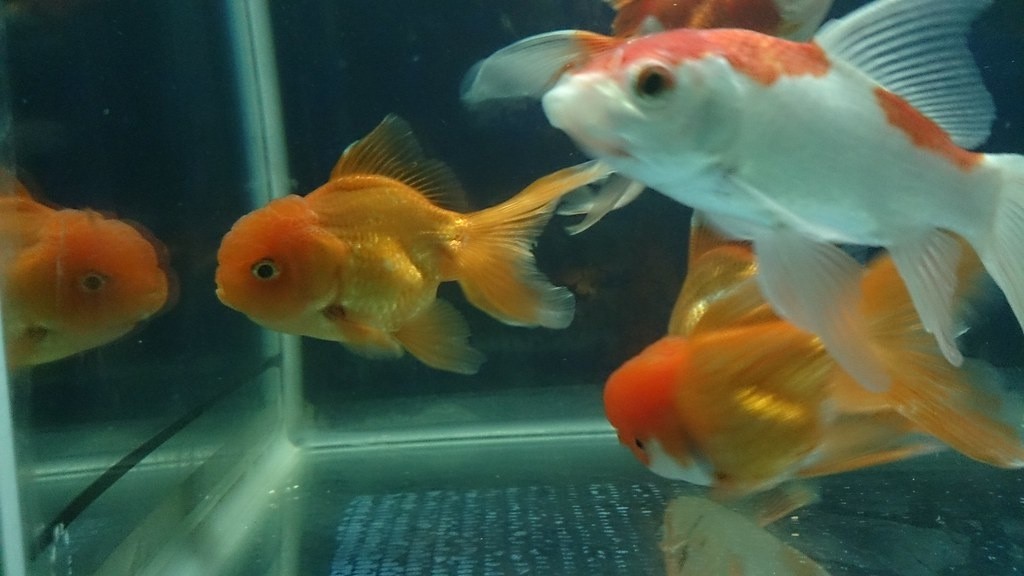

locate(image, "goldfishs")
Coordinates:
459 0 1023 576
0 166 170 368
215 112 618 373
